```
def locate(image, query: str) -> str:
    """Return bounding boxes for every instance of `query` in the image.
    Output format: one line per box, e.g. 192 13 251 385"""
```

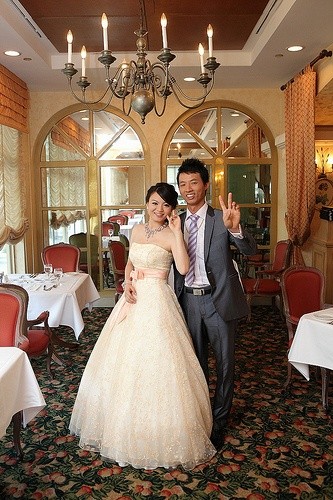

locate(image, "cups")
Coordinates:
127 214 131 220
117 219 121 226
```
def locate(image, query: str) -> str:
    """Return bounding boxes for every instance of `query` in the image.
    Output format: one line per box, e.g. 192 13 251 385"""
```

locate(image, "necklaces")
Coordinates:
145 221 168 241
185 219 204 234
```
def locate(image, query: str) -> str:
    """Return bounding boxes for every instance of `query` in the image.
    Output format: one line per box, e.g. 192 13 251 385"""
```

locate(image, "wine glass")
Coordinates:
108 229 113 239
54 268 63 287
0 271 4 285
44 263 52 281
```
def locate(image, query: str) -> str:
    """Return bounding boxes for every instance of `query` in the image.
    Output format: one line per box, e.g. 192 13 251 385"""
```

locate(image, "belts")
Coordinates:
183 286 212 296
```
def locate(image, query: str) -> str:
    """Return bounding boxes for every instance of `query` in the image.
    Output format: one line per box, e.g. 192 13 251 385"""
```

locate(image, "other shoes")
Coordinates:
210 427 225 451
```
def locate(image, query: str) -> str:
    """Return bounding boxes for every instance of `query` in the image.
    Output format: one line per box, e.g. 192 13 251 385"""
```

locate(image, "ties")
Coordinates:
187 215 200 286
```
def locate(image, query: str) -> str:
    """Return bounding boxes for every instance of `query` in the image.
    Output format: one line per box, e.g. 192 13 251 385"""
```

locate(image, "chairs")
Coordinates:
40 242 80 273
0 284 69 378
69 210 139 305
241 216 292 323
281 266 333 389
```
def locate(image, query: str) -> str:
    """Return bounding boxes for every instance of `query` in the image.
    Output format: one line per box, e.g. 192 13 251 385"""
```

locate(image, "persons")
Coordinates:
171 158 258 452
68 182 216 470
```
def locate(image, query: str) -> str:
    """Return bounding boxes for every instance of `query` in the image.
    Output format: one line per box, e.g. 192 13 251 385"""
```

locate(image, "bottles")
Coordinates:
103 238 108 248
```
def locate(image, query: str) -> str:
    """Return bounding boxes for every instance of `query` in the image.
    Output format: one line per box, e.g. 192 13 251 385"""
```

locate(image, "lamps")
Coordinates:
62 0 221 124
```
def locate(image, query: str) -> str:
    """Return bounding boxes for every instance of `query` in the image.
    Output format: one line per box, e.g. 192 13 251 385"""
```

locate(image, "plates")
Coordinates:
313 178 333 210
6 274 53 289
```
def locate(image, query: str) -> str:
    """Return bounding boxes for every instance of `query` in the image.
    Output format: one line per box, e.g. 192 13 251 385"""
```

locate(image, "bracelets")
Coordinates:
122 281 132 288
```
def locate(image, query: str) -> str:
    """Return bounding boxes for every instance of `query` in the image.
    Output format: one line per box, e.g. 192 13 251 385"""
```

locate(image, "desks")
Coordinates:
120 225 134 240
288 308 333 415
128 218 143 225
0 273 101 348
102 236 120 258
230 245 270 261
0 347 47 461
134 214 144 218
